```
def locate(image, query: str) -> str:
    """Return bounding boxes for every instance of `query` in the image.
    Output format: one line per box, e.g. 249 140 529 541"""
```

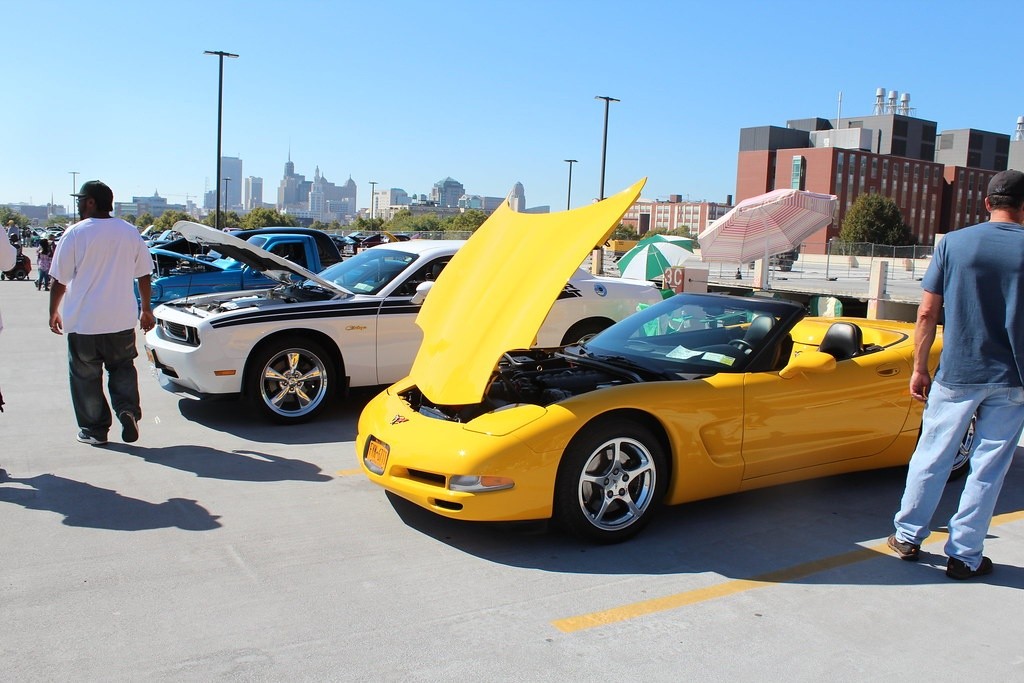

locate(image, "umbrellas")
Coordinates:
615 235 695 288
697 189 836 290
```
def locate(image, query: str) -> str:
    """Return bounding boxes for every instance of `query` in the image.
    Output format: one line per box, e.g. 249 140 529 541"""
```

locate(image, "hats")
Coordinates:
7 220 14 224
987 169 1024 197
70 180 113 202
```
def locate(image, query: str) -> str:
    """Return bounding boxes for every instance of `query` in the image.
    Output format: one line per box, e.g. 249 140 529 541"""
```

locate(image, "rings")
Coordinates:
50 327 54 329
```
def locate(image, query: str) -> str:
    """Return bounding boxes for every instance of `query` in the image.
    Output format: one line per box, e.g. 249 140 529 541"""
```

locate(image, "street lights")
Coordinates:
69 172 80 223
595 96 620 243
204 52 239 227
222 178 232 226
564 160 579 210
370 182 380 219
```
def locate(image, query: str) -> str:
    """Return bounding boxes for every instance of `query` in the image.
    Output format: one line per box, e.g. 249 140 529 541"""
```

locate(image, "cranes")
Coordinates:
162 193 197 199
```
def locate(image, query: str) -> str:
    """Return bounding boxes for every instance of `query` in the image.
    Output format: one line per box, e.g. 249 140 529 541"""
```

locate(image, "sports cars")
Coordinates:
352 176 986 546
144 220 681 424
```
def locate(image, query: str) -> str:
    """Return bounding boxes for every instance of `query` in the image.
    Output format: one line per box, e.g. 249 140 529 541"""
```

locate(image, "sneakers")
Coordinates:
946 556 993 580
887 532 921 560
119 411 139 443
78 431 108 445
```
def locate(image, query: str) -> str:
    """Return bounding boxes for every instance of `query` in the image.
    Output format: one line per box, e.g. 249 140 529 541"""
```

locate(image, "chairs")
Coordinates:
737 314 780 370
817 322 864 362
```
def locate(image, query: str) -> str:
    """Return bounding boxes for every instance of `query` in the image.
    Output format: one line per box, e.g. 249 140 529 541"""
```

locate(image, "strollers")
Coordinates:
0 234 32 281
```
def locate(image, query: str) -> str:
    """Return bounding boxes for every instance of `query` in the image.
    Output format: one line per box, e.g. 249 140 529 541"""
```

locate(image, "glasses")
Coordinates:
76 197 88 204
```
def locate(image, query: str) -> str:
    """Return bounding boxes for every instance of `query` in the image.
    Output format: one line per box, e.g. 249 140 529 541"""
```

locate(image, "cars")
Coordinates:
18 225 65 245
134 226 407 323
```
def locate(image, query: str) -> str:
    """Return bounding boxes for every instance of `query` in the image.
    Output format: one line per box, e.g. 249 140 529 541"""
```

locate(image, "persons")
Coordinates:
48 180 156 443
887 169 1024 583
0 219 56 412
